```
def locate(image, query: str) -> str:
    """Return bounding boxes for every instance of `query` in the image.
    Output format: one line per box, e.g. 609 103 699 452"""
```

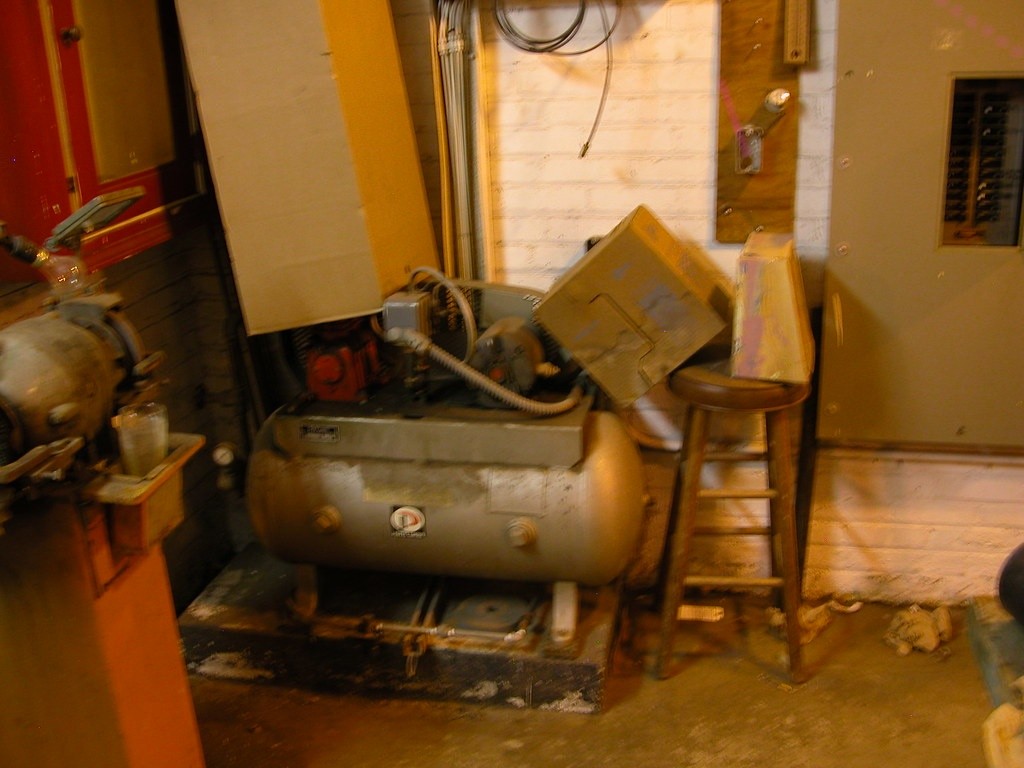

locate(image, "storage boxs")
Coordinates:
532 206 735 411
731 231 815 384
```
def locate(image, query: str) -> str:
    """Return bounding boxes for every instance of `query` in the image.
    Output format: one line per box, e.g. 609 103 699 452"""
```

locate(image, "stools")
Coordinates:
653 343 814 688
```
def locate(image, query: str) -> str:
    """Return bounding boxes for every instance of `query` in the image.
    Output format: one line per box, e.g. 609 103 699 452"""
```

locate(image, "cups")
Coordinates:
117 402 171 477
